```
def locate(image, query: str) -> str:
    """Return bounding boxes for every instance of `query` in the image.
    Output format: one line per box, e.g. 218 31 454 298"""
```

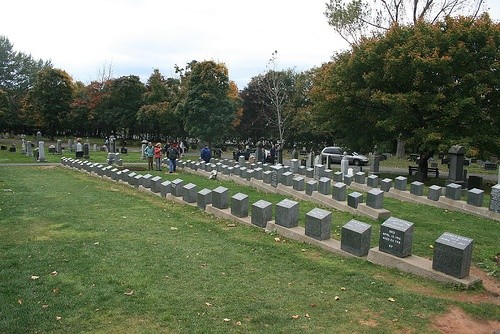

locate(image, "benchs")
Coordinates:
408 165 439 178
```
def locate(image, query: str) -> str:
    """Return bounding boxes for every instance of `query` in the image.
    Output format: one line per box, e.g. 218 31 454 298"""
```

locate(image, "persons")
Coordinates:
416 155 421 164
245 143 250 160
236 137 274 151
163 142 171 156
154 142 165 170
214 145 225 159
144 142 155 170
235 147 240 161
202 145 212 164
169 143 180 172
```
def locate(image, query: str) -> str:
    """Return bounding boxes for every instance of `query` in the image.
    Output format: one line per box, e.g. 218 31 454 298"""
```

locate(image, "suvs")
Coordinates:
319 145 369 166
